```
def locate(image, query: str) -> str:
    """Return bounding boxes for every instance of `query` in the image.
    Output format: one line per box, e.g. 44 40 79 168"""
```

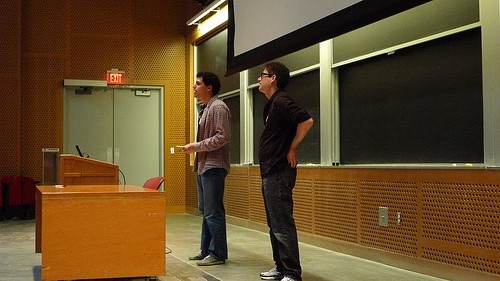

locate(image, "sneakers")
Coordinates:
189 250 205 260
260 266 282 279
280 275 296 281
196 254 223 266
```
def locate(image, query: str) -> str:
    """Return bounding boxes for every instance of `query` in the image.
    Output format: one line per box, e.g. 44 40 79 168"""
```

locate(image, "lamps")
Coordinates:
186 0 227 26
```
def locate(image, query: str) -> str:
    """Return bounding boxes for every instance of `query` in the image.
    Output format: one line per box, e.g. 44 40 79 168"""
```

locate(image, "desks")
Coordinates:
35 185 167 281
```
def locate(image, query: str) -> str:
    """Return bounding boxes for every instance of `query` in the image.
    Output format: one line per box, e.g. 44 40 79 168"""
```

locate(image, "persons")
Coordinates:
182 72 232 266
257 62 314 281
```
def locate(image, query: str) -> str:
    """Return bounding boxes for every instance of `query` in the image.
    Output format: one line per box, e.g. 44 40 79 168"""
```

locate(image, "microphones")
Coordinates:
75 144 83 157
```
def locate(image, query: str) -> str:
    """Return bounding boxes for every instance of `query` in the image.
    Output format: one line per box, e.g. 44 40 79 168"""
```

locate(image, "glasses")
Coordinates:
261 72 273 80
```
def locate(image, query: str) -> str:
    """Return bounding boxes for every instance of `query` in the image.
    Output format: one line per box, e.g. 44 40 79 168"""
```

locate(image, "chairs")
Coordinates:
0 175 42 219
143 175 165 189
